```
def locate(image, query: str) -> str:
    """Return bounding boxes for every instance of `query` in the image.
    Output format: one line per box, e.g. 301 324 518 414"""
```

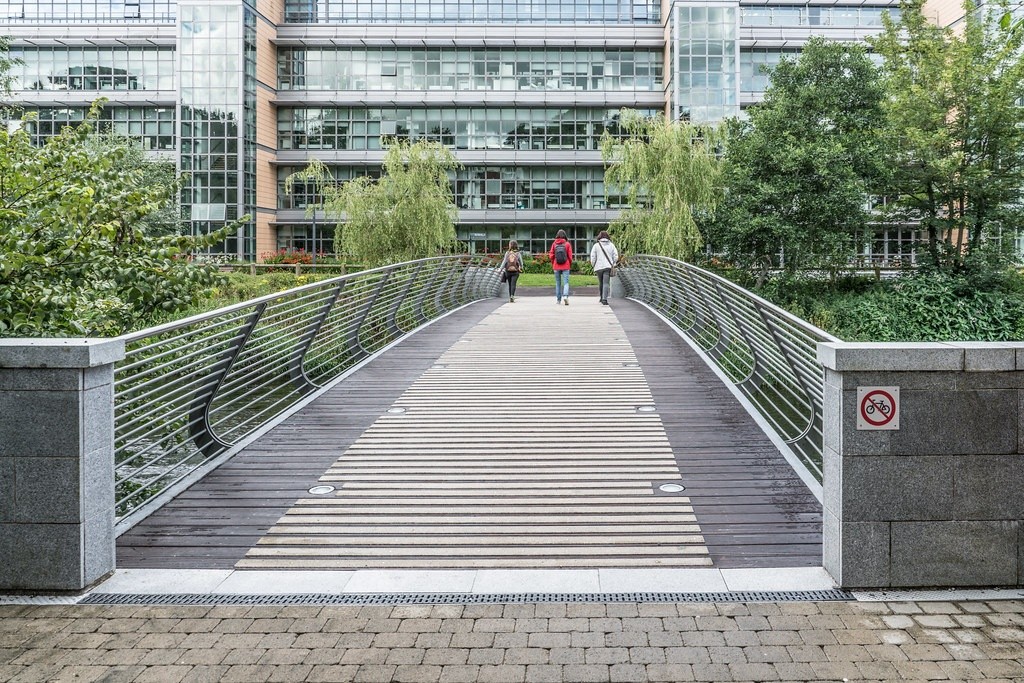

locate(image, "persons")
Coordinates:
550 230 572 305
591 231 618 306
498 241 523 303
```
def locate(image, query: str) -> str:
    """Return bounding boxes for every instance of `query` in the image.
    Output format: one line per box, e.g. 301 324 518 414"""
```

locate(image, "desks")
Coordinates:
546 145 573 150
428 145 455 149
299 203 333 208
610 204 643 208
622 86 649 90
611 145 634 149
488 204 515 209
520 142 543 150
488 145 515 149
100 79 112 89
547 203 574 209
299 144 333 149
429 86 452 90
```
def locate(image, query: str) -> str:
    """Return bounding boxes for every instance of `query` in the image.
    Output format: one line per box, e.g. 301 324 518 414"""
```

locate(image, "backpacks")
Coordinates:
555 243 567 264
506 251 521 271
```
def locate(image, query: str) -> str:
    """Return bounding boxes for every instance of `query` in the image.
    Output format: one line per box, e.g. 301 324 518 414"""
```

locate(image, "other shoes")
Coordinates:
564 296 569 305
602 300 609 307
599 299 602 303
510 295 515 302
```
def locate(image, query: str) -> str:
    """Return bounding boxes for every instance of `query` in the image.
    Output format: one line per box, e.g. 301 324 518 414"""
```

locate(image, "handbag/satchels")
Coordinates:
501 272 507 283
610 266 617 276
